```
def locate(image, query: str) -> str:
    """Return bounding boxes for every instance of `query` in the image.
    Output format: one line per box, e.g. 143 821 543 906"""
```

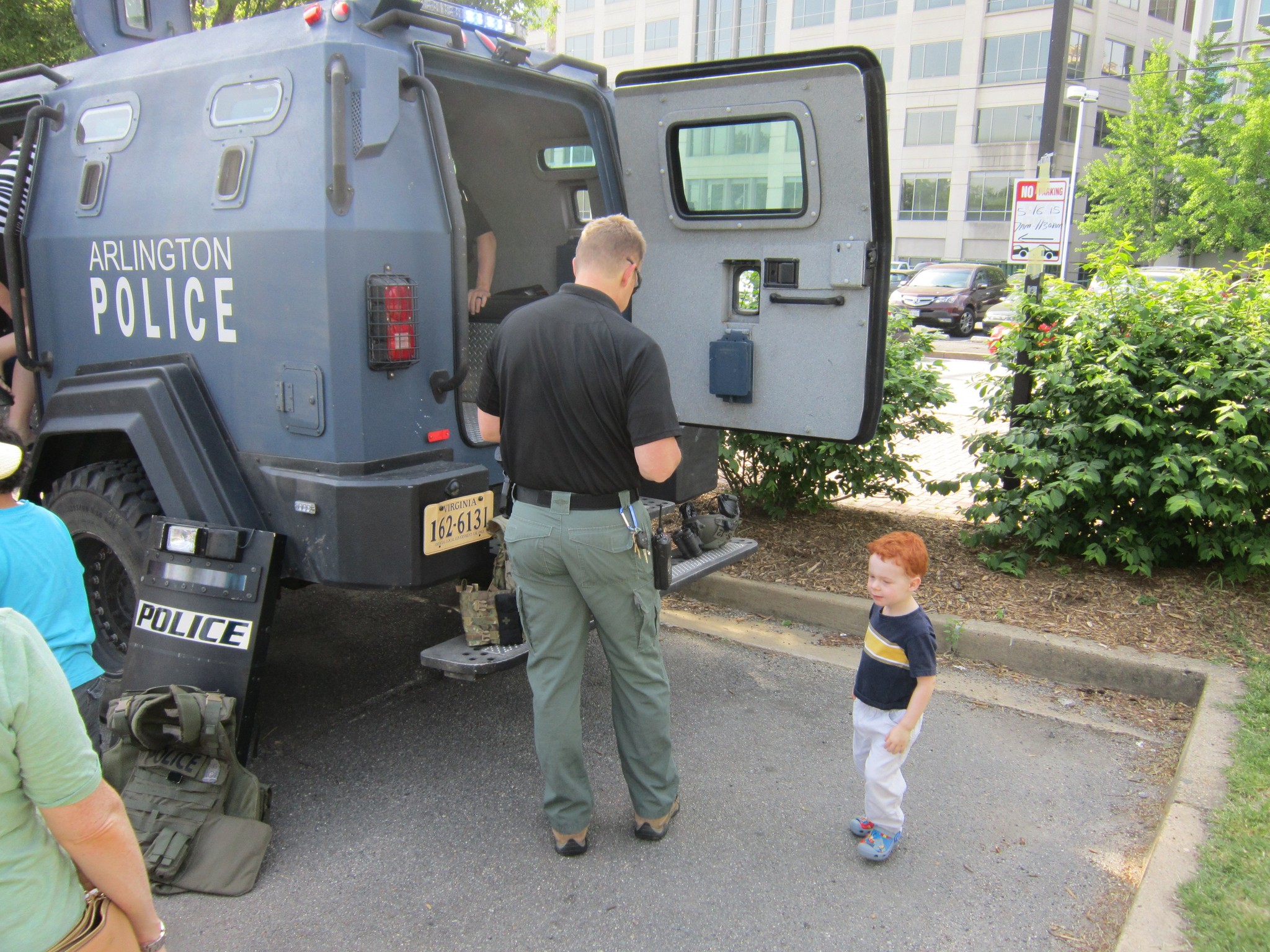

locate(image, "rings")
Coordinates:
476 296 482 300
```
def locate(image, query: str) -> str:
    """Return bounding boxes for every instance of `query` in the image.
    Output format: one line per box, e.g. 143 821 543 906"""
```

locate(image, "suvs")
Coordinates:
982 292 1031 342
892 262 1007 335
1006 267 1270 328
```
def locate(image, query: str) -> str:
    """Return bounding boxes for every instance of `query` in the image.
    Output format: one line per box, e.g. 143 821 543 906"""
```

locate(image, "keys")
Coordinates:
630 526 651 563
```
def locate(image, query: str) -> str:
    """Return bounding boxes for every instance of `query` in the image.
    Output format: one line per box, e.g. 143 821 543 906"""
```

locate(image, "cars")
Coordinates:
891 261 910 272
888 268 918 298
913 262 937 271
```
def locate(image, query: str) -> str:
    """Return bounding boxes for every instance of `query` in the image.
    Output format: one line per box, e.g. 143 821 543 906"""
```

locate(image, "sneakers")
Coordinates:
860 827 903 863
551 825 593 855
850 814 881 841
634 795 680 838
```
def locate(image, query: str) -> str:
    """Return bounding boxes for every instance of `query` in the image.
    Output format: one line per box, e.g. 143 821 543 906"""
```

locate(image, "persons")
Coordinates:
454 179 497 316
842 531 938 862
0 423 112 774
0 606 172 952
0 119 42 449
476 212 691 856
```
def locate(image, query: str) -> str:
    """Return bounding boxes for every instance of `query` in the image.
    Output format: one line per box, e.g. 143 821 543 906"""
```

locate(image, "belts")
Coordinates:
512 481 640 511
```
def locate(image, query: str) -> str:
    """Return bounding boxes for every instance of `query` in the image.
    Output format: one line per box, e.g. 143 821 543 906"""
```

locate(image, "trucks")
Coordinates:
0 0 899 737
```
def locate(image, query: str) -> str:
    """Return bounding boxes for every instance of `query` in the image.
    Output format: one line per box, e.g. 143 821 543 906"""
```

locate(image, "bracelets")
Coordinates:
140 921 166 952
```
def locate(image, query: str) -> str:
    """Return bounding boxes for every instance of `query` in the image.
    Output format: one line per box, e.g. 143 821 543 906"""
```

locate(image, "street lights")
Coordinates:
1054 85 1101 282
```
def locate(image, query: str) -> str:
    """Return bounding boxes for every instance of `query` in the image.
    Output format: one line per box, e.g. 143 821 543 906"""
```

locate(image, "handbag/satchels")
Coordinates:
42 891 168 952
